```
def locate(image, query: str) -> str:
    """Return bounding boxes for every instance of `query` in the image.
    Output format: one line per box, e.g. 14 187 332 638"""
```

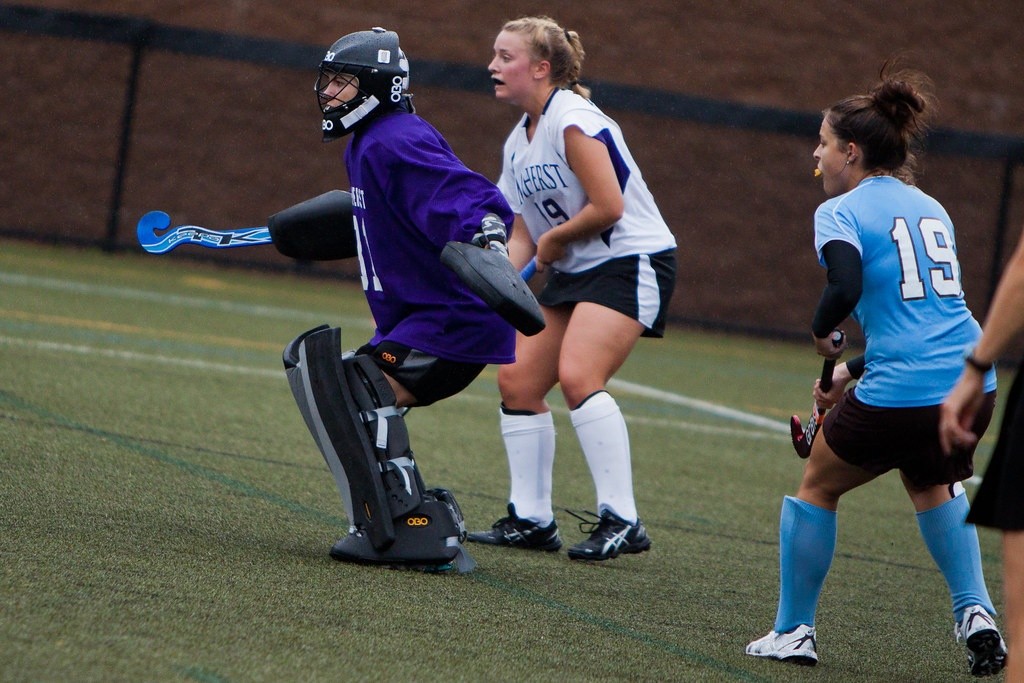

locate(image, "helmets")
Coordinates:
314 26 417 144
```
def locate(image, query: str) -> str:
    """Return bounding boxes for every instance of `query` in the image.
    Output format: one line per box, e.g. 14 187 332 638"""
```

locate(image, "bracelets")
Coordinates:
963 350 990 373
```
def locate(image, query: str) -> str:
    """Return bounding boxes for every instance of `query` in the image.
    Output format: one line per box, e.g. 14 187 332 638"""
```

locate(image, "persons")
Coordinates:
941 232 1024 683
468 17 677 561
744 76 1007 673
269 30 517 560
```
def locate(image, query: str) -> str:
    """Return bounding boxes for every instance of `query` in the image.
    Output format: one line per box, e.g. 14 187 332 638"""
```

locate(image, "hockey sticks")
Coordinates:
788 327 846 461
137 210 277 256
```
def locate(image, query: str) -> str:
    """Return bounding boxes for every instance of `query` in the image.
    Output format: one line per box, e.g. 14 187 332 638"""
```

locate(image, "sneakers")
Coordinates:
564 509 653 561
954 604 1008 677
467 502 563 553
330 488 478 575
746 624 819 666
